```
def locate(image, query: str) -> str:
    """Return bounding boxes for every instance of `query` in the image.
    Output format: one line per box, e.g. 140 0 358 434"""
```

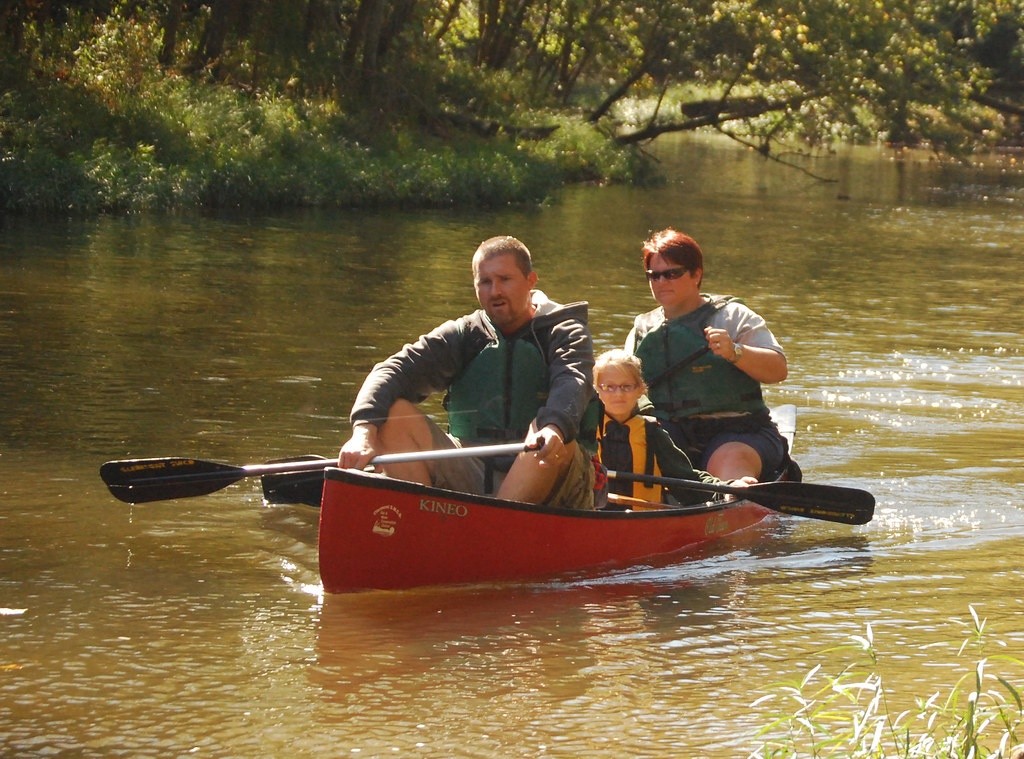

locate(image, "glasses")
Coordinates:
598 382 638 392
646 267 688 280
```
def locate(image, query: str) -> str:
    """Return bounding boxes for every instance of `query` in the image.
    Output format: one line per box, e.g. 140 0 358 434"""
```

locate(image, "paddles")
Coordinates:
604 493 676 509
98 432 547 505
258 454 376 506
605 470 877 525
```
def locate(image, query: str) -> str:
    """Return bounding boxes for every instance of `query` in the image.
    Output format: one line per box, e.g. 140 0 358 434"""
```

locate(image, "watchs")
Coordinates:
726 343 743 363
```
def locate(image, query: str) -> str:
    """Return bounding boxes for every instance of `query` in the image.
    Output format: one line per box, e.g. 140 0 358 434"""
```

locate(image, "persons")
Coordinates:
338 230 788 509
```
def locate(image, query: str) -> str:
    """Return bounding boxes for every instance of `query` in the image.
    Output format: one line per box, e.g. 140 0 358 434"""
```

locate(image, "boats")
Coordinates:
316 403 798 594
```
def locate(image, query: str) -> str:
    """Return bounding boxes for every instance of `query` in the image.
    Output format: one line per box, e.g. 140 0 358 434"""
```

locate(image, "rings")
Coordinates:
716 343 721 349
